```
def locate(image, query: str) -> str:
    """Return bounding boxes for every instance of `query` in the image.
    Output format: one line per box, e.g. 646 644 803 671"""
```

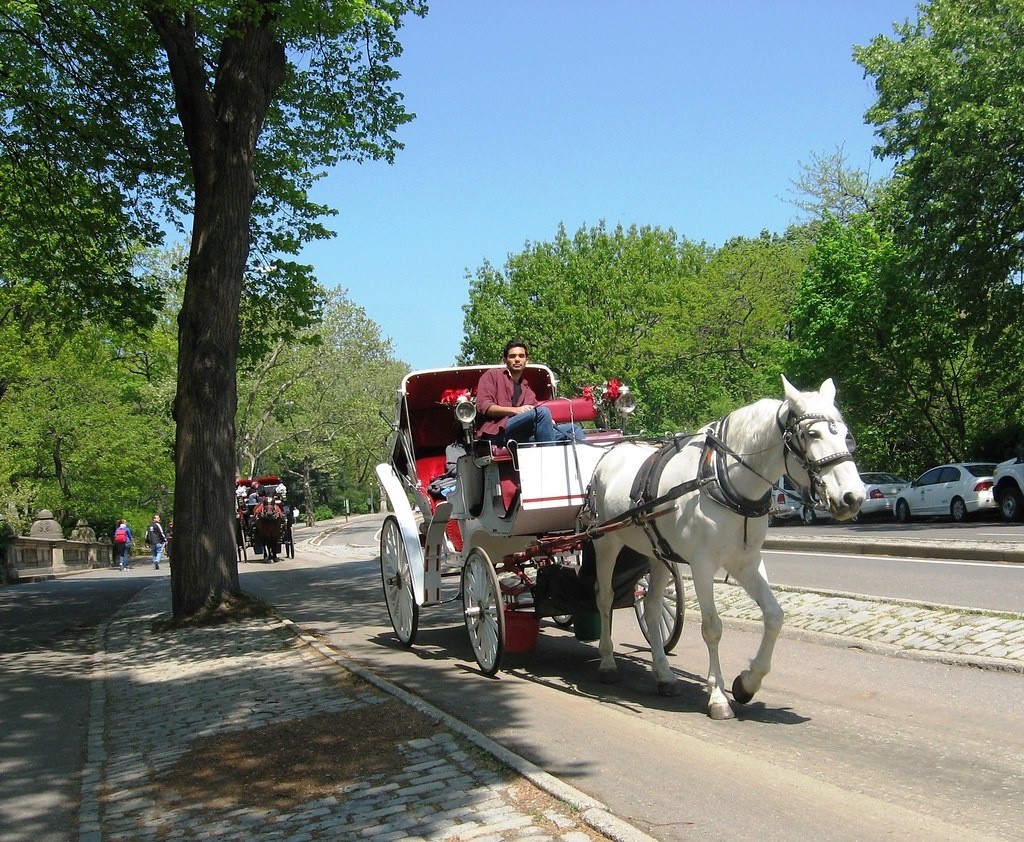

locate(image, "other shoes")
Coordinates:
155 563 159 570
119 563 123 571
124 568 126 571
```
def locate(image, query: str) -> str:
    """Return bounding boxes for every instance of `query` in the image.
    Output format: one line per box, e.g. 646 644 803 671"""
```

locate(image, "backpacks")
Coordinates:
115 527 127 542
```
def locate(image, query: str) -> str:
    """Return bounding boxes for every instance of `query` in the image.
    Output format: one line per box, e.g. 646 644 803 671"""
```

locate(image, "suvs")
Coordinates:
768 474 802 525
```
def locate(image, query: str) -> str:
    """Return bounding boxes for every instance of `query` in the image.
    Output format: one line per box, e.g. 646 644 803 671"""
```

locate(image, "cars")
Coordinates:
993 458 1023 520
800 472 911 524
893 461 998 522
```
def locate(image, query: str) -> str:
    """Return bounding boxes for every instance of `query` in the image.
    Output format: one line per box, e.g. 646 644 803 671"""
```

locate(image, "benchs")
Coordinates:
414 447 448 502
245 485 278 494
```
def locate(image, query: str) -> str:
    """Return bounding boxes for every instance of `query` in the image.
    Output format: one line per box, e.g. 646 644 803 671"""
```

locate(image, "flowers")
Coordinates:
440 388 473 407
581 378 623 429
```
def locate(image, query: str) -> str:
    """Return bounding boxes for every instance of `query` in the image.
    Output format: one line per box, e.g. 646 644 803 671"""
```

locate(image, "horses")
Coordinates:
252 495 285 564
579 371 867 721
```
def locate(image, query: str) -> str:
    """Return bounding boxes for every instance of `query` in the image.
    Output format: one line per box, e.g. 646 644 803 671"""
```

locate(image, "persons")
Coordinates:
165 521 175 568
144 514 168 570
292 505 300 525
112 517 136 572
473 337 587 447
249 482 267 504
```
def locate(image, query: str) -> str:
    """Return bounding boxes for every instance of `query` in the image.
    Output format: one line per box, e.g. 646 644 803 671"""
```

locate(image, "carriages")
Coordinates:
236 477 297 563
375 363 871 718
